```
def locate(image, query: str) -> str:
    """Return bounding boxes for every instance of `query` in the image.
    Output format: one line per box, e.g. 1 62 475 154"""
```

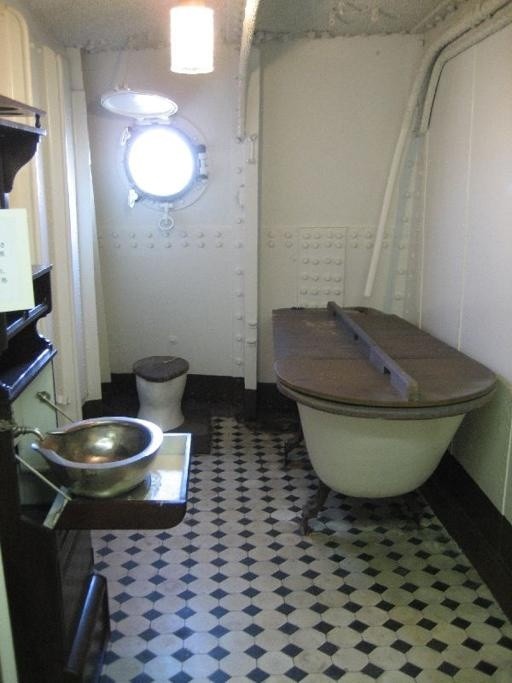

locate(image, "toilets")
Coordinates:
133 356 189 433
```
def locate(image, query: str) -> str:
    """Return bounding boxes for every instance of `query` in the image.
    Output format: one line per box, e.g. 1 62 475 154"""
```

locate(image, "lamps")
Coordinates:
170 5 214 75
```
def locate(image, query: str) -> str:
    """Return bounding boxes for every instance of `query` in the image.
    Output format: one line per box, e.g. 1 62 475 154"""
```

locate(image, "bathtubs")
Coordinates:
273 305 498 535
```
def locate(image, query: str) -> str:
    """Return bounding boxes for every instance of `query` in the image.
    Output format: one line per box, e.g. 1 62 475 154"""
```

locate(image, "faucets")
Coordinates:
12 425 46 442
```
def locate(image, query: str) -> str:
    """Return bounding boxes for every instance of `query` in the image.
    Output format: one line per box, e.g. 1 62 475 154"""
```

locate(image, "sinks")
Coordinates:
31 415 164 499
